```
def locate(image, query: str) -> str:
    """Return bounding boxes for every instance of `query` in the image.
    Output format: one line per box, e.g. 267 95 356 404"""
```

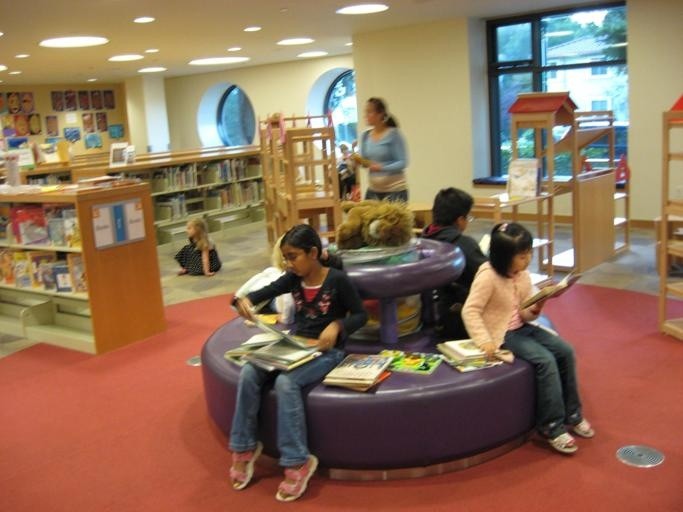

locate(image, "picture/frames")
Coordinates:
108 142 128 168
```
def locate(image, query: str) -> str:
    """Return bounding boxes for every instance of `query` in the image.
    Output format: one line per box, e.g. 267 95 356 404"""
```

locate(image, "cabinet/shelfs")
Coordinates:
462 90 631 292
78 153 265 240
653 212 682 277
409 202 434 237
0 144 256 187
656 110 683 343
256 114 344 250
0 181 166 357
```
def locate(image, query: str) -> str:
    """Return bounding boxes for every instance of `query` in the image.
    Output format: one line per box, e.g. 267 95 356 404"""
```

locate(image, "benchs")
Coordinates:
196 310 554 480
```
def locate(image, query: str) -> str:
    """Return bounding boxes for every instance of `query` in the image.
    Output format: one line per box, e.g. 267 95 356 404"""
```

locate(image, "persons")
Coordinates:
0 174 88 294
461 222 594 453
421 188 489 278
352 98 409 203
175 217 221 275
228 223 368 502
338 144 355 194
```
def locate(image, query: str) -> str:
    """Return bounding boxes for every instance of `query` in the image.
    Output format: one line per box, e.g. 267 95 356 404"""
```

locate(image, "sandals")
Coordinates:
228 440 263 490
275 454 318 502
569 417 594 437
545 431 578 454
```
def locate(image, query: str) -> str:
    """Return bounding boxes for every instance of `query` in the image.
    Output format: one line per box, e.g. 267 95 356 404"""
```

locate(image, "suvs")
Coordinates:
515 121 629 188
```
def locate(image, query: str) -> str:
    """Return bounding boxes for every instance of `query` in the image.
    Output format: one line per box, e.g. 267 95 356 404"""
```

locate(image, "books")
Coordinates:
517 271 583 313
377 349 443 376
224 330 395 393
436 338 505 373
137 158 264 220
